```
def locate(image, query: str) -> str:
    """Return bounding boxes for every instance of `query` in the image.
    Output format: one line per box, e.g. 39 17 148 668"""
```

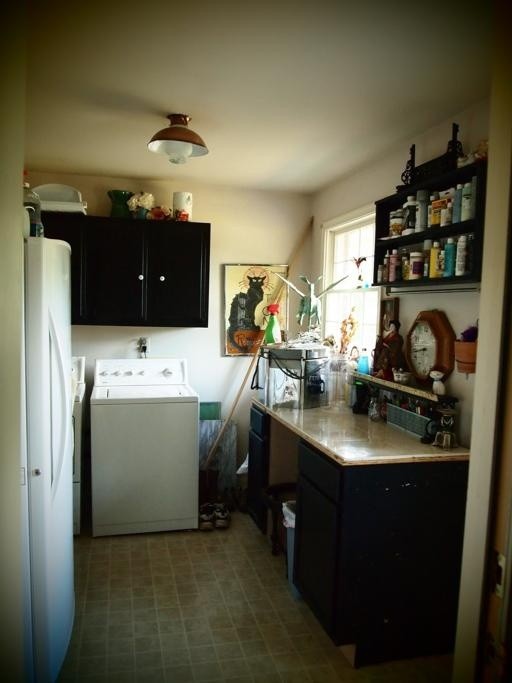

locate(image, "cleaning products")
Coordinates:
266 304 281 345
376 181 476 284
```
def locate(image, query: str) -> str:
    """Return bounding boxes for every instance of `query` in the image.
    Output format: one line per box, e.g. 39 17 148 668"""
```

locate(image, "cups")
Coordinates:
171 190 193 222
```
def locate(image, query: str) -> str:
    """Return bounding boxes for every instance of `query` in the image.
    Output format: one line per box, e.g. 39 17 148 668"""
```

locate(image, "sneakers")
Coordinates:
199 503 230 531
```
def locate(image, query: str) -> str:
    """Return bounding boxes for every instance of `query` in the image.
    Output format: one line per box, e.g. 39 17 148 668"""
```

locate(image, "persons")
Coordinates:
373 316 404 379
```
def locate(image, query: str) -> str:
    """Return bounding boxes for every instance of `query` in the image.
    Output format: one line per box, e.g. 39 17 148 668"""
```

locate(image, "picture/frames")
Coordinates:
224 263 289 356
380 297 399 324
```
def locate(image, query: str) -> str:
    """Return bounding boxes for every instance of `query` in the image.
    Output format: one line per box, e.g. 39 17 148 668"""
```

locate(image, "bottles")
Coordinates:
358 346 369 374
377 177 472 284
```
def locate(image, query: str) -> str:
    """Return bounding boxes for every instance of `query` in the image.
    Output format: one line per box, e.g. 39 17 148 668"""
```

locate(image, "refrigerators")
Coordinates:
24 236 75 682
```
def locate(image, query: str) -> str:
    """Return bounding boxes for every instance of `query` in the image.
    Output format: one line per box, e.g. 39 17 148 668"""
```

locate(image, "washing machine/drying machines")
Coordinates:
70 357 87 536
90 360 200 537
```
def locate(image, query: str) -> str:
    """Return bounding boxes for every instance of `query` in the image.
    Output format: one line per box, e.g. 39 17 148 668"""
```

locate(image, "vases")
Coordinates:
137 207 148 219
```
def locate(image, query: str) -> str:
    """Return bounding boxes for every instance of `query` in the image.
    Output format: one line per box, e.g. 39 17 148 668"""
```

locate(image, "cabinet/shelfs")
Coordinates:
40 210 210 327
372 122 488 287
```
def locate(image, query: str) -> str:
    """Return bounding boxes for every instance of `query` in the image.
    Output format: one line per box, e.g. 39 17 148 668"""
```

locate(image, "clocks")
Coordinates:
406 310 456 383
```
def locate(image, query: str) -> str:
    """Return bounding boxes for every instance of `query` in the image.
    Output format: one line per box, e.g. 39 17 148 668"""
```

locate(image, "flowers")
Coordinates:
126 192 154 210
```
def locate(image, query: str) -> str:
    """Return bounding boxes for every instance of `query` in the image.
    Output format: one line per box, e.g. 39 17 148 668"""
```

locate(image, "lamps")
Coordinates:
147 114 209 165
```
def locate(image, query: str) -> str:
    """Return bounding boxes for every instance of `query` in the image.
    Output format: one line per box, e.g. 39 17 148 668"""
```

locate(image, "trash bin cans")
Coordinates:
282 500 303 601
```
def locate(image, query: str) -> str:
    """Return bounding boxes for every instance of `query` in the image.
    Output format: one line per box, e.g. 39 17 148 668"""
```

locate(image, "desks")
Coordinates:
247 397 469 667
